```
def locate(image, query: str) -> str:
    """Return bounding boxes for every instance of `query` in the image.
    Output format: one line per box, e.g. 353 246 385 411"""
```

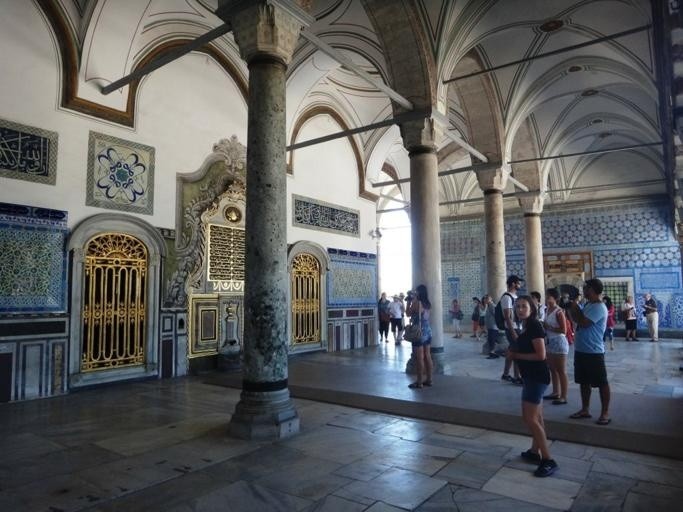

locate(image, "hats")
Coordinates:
584 278 604 294
393 295 401 301
506 274 525 284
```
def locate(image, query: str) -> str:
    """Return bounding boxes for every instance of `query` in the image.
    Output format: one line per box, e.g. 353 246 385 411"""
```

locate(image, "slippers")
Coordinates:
596 417 612 425
542 394 561 400
551 399 568 405
570 412 593 419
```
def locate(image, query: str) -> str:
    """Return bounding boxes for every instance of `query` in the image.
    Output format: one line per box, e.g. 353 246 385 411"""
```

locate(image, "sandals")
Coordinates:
423 380 433 387
408 382 425 389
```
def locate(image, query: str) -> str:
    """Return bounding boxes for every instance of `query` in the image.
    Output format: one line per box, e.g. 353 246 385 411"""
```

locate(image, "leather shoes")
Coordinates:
611 347 614 350
649 339 658 342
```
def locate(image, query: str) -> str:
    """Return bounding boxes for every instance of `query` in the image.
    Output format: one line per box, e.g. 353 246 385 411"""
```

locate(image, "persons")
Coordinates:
558 290 575 345
529 291 545 322
501 295 560 477
564 277 612 425
573 293 586 311
387 294 404 345
403 284 435 389
483 295 506 360
542 288 569 406
602 295 616 351
470 296 482 337
494 275 525 385
476 297 489 341
621 296 639 342
399 292 405 340
378 292 391 342
640 293 659 342
448 299 465 338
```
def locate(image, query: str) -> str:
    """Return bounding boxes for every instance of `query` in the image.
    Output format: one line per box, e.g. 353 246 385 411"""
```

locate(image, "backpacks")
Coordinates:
494 291 515 331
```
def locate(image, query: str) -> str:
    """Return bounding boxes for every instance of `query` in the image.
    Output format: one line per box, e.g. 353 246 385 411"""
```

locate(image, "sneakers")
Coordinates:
534 459 559 477
511 376 524 385
521 449 541 463
501 374 517 382
632 338 639 341
625 337 630 341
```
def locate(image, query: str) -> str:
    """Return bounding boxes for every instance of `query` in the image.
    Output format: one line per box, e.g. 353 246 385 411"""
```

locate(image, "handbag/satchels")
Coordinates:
404 299 423 343
